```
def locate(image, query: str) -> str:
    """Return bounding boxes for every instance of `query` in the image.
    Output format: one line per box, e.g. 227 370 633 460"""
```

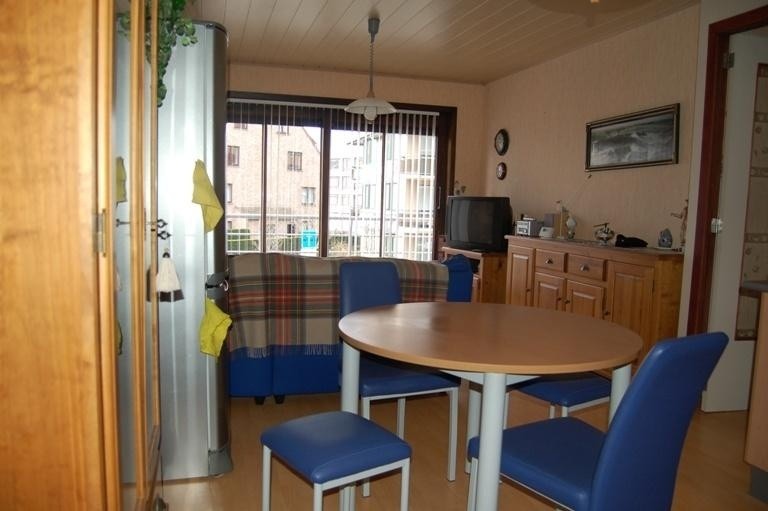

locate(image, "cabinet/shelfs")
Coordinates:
504 234 684 380
441 247 507 304
0 0 167 511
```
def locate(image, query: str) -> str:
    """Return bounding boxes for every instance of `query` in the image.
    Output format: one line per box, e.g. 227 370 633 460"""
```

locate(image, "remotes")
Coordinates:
523 216 537 221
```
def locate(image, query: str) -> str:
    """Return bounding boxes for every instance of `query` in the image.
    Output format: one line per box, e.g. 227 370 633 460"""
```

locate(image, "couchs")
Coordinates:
228 253 473 405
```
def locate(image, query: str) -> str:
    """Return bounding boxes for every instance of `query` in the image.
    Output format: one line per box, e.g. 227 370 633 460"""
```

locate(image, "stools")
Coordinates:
499 371 611 484
261 410 411 511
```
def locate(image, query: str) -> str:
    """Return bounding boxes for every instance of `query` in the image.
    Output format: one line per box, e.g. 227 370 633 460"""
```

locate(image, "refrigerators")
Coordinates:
154 20 232 486
113 10 153 486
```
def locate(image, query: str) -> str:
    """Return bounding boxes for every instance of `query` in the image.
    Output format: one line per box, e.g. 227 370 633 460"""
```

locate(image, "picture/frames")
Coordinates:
585 102 680 172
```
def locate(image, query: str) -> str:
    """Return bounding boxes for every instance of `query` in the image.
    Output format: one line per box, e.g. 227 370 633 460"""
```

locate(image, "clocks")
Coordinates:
494 129 509 155
496 162 506 179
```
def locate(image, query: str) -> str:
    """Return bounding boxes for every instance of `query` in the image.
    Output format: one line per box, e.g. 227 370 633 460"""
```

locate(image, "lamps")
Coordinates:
344 17 397 121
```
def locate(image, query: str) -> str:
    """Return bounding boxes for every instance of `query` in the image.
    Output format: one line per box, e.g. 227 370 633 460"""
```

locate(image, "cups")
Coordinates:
566 218 578 241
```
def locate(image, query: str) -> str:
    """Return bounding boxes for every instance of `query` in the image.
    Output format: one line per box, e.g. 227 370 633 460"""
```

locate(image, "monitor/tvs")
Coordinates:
445 196 513 252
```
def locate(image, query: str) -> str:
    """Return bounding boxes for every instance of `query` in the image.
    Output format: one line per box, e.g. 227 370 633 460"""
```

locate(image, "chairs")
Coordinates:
338 261 459 497
467 331 729 511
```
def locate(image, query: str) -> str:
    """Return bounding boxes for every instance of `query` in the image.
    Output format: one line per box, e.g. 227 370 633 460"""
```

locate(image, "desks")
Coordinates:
337 302 643 511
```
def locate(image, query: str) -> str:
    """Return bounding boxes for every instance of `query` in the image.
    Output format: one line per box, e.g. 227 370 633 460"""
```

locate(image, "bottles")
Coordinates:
658 228 672 248
